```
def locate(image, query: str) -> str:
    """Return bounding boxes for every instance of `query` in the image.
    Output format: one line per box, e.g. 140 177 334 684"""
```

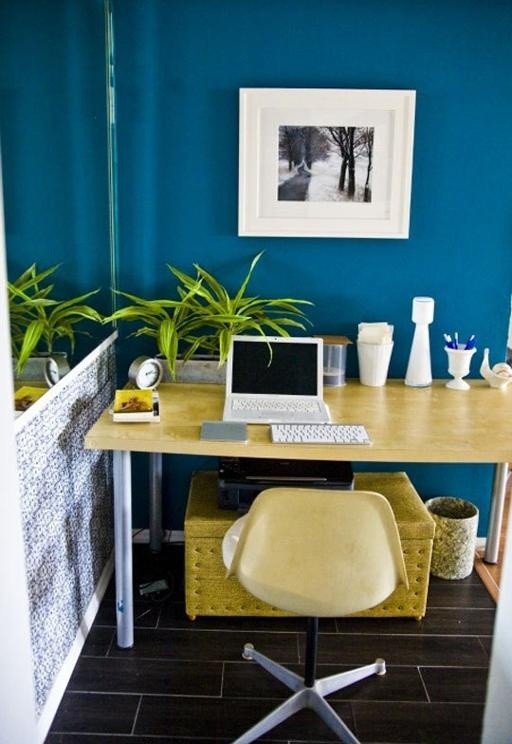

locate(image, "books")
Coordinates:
16 385 49 410
108 390 160 423
113 389 154 423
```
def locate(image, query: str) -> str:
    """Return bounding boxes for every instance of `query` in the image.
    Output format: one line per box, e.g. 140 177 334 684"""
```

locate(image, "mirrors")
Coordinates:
4 0 119 437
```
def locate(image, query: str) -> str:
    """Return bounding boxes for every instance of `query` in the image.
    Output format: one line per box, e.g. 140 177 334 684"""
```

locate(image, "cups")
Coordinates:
357 341 394 388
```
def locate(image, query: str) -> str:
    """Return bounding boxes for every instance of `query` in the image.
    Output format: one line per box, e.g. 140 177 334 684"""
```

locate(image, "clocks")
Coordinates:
127 356 164 391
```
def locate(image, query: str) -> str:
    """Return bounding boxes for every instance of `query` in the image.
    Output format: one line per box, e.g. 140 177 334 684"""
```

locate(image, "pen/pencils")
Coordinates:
443 332 459 350
464 335 477 350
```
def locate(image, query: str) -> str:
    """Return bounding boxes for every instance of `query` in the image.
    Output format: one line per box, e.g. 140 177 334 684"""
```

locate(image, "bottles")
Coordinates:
404 296 434 390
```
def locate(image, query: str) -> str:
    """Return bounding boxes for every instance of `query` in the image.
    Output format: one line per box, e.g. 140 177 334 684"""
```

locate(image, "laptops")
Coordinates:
222 334 329 424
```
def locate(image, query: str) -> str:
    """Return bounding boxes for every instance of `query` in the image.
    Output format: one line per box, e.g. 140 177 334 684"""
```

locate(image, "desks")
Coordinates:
77 375 511 652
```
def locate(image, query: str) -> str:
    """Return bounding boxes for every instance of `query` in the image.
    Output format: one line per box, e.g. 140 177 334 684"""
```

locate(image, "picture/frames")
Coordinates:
234 86 415 243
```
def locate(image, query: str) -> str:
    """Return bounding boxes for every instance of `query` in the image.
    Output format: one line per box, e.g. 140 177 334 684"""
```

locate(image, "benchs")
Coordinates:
182 466 438 625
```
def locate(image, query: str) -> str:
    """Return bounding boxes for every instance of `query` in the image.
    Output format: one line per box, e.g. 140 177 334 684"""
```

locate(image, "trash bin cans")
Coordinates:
424 496 480 581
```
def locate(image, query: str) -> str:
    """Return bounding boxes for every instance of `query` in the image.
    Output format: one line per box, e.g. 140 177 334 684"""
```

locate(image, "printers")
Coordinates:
217 457 354 516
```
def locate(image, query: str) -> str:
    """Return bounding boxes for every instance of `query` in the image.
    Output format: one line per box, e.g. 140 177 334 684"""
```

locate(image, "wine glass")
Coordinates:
443 345 476 391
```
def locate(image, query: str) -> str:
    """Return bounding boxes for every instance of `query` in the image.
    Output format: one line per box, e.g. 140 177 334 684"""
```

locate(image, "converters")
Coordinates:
139 579 168 596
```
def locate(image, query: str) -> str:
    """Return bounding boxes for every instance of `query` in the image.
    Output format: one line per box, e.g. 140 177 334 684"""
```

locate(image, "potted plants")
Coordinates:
105 249 319 389
5 264 103 380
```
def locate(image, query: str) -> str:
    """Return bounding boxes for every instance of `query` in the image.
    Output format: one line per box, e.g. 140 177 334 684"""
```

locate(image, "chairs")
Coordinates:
217 486 412 743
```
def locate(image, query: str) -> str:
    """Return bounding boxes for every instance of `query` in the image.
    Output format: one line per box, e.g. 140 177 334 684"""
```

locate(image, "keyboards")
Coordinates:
271 424 371 445
